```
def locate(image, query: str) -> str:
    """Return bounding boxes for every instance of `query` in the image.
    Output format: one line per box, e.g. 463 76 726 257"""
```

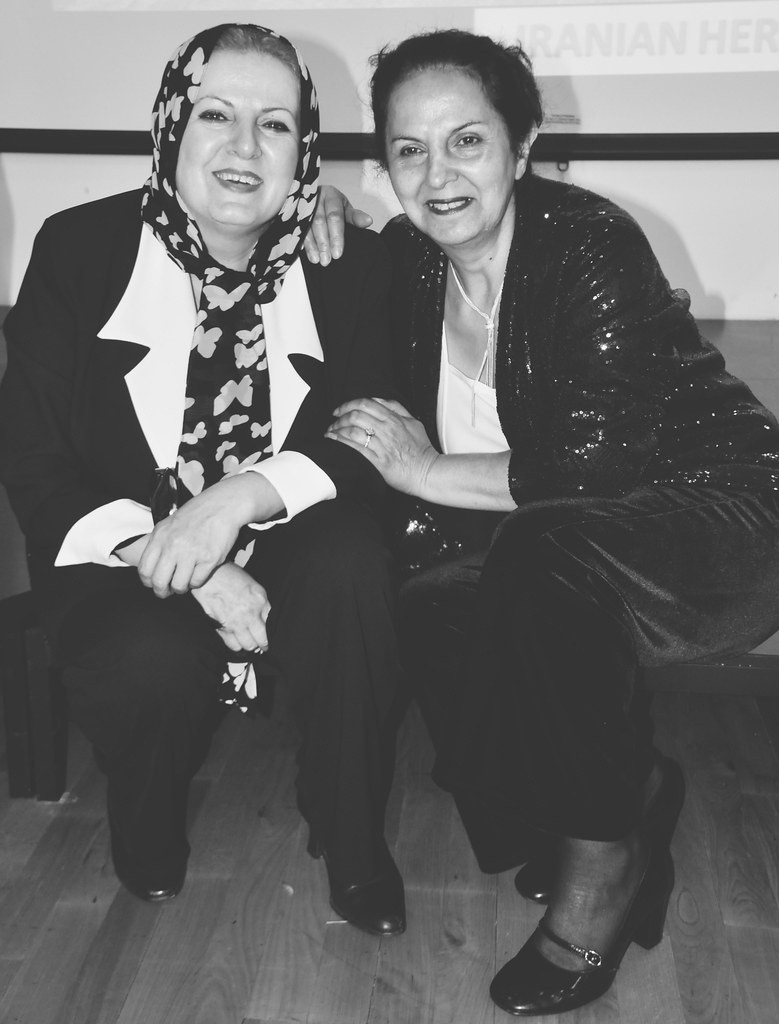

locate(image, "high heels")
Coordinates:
489 841 675 1016
514 755 687 905
306 819 406 937
104 797 190 902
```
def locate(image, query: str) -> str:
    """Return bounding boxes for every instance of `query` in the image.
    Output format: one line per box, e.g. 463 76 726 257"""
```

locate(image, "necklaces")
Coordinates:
447 262 504 431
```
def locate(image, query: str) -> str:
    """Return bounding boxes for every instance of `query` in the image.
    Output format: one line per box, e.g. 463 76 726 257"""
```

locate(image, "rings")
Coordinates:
365 429 374 448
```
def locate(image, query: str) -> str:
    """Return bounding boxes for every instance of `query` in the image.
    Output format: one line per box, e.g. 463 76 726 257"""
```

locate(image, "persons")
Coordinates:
0 22 414 936
303 32 779 1016
